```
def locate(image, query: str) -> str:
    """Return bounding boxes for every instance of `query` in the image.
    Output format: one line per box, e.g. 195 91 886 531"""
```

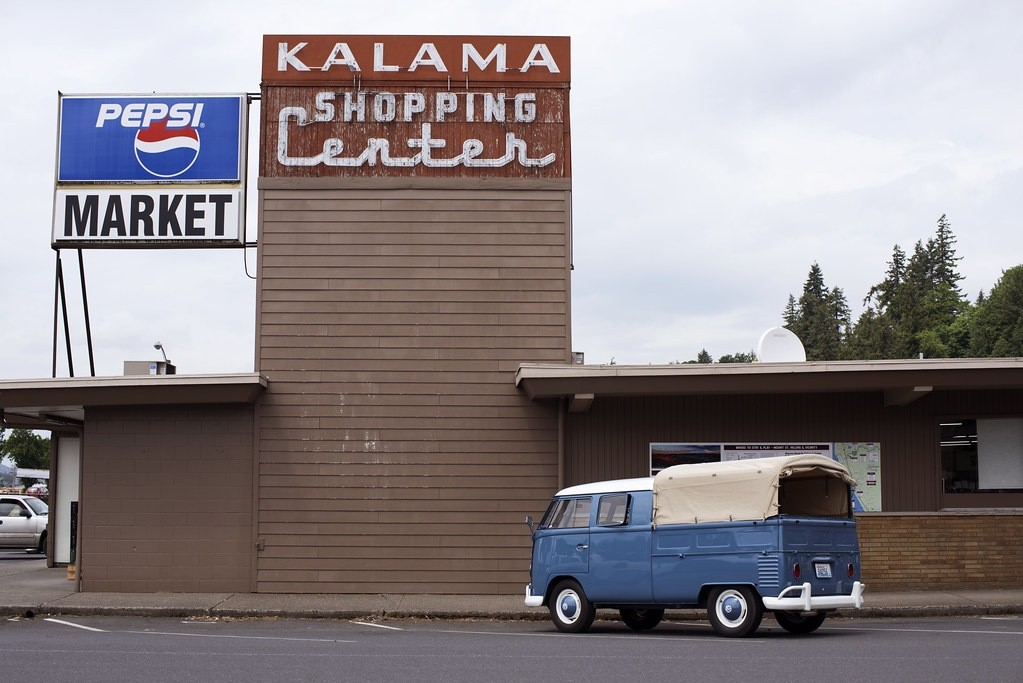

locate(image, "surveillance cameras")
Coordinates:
154 342 162 349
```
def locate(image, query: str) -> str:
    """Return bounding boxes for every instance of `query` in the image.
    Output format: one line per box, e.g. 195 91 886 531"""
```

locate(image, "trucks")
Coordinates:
524 453 866 638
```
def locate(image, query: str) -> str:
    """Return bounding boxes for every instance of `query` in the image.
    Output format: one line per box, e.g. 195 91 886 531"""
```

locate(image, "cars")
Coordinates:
0 495 49 556
